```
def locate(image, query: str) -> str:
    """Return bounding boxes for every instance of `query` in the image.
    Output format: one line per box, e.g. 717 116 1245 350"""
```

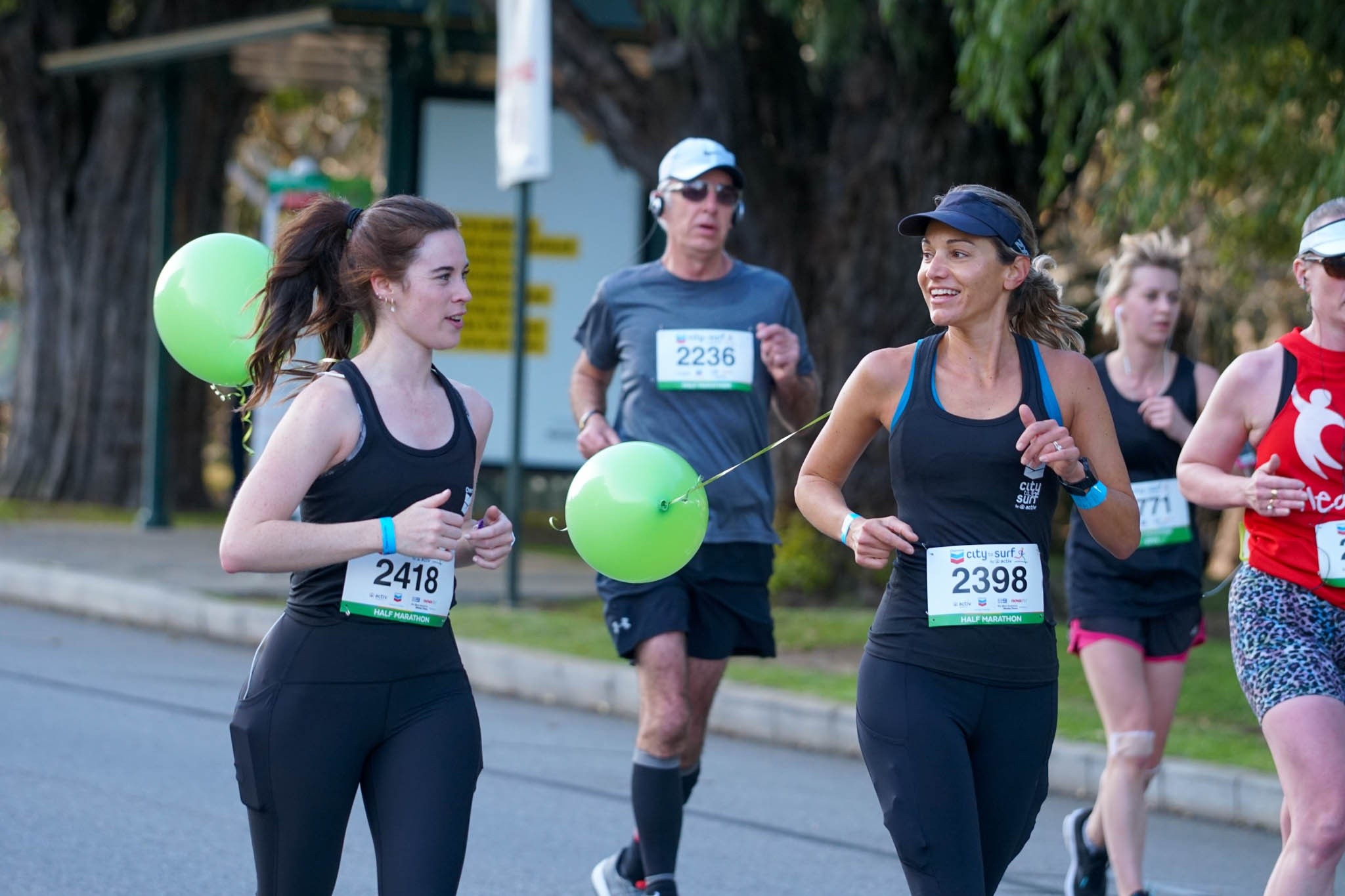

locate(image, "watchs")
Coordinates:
1058 456 1099 496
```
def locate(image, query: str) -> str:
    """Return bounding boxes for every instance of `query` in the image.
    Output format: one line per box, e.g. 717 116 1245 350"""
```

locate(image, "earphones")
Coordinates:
1303 276 1307 290
1115 304 1123 319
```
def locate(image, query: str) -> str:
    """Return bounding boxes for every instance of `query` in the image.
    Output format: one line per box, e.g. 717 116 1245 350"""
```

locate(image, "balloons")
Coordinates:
152 231 290 387
565 439 708 584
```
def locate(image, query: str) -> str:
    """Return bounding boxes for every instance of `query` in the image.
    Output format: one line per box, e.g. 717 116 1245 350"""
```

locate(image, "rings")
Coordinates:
1053 440 1063 451
1271 489 1277 498
1267 499 1275 510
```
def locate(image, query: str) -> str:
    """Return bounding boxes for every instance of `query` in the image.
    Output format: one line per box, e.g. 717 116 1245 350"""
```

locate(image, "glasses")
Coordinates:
666 179 738 204
1302 254 1345 280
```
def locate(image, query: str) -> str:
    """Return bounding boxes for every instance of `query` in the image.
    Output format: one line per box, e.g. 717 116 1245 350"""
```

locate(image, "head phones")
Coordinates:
648 190 745 227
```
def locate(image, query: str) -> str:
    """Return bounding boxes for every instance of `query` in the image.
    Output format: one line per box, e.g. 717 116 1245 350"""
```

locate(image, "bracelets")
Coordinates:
579 408 604 428
1070 479 1108 509
381 515 396 556
840 512 860 543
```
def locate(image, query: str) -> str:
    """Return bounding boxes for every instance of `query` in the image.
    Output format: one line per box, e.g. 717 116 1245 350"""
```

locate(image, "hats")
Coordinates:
1297 218 1345 257
659 137 745 191
896 190 1032 266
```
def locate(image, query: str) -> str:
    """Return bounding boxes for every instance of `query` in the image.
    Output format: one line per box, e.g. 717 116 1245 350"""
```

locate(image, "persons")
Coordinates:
216 194 517 895
567 136 820 896
1060 230 1221 896
1175 194 1343 895
794 184 1143 896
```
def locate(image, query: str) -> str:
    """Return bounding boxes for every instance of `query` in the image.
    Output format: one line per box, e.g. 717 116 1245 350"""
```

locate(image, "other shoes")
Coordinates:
1062 808 1108 896
591 847 646 896
